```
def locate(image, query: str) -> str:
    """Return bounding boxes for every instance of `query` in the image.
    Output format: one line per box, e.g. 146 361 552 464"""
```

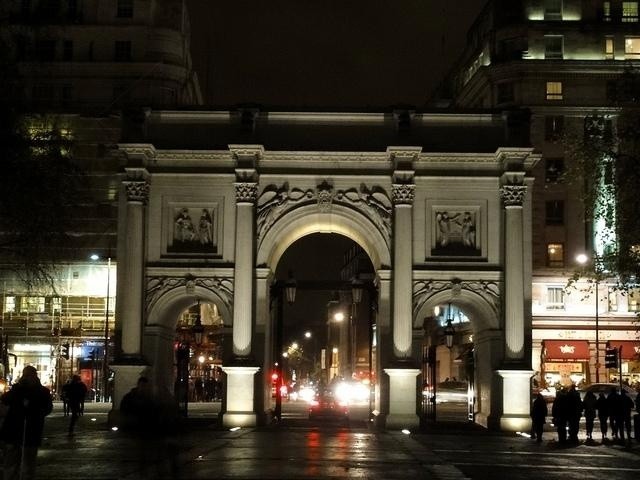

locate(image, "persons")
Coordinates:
437 212 473 250
0 365 53 480
530 375 640 445
115 374 223 480
339 190 392 239
172 207 214 245
255 188 311 240
60 372 87 443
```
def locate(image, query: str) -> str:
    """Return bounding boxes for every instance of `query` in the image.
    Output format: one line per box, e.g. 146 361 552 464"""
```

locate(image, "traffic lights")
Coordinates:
61 344 69 360
605 349 620 369
89 349 94 361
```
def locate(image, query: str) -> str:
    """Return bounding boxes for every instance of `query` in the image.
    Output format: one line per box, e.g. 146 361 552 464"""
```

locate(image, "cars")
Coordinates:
577 383 639 410
424 383 468 405
272 380 369 423
61 388 100 402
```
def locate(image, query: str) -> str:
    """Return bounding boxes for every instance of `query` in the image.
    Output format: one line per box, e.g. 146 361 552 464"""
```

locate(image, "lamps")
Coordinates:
444 302 456 349
192 299 204 345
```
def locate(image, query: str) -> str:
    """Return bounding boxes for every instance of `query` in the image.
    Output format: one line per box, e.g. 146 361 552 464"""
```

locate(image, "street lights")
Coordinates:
575 254 600 380
353 277 373 422
90 248 110 396
274 278 297 422
